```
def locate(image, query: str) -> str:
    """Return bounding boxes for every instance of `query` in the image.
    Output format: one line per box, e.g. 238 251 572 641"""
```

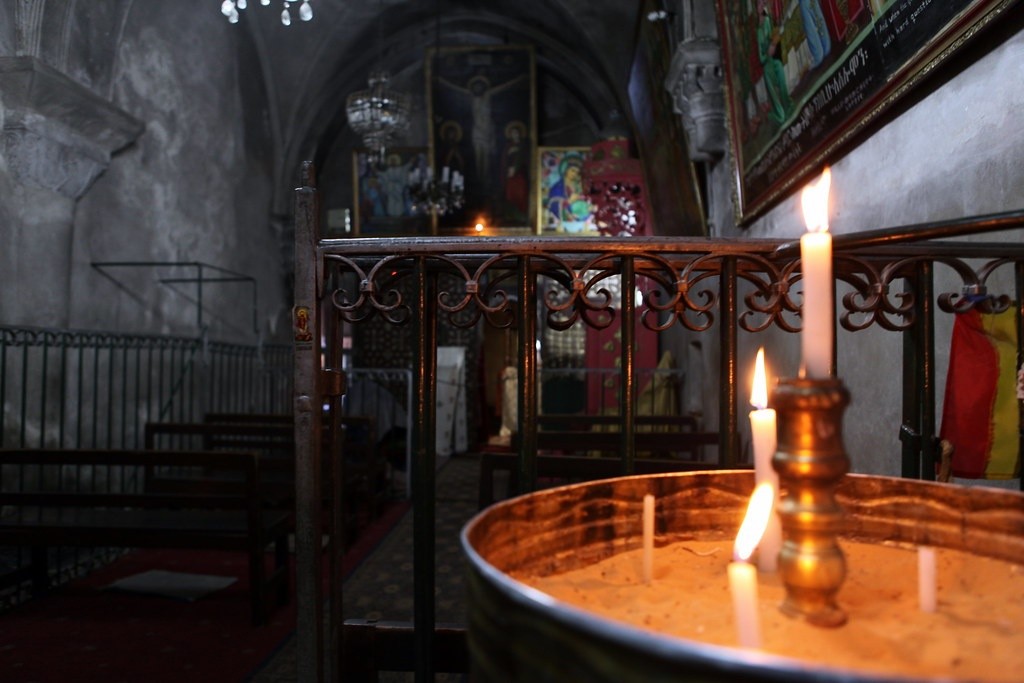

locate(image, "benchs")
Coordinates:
0 447 293 625
480 414 743 515
142 410 408 570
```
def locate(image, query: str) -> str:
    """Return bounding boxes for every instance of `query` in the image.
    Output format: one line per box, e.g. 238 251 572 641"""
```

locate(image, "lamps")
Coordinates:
345 0 411 175
405 166 464 217
221 0 314 26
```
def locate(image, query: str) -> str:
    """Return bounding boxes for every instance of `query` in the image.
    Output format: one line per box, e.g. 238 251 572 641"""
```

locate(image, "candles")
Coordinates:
643 494 655 584
800 230 836 382
916 544 938 612
749 408 783 579
728 563 759 649
